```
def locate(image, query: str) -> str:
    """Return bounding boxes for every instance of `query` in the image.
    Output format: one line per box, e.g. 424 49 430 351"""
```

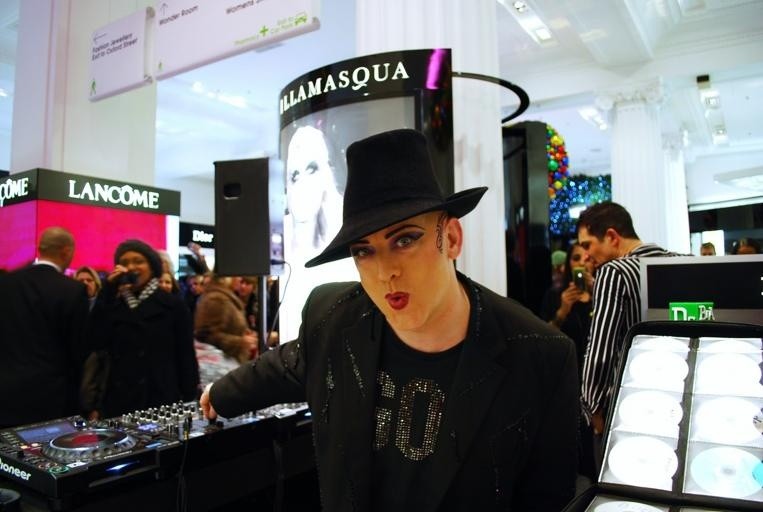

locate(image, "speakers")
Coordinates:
214 158 270 276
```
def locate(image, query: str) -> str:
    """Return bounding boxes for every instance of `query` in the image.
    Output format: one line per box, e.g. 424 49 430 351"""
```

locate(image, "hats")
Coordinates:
115 239 162 279
305 128 489 268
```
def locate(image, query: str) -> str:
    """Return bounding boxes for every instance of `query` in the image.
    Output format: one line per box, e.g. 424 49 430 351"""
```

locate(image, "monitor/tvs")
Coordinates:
503 121 558 320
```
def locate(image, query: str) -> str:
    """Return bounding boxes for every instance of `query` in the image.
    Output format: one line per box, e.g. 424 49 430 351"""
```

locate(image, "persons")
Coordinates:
199 129 579 512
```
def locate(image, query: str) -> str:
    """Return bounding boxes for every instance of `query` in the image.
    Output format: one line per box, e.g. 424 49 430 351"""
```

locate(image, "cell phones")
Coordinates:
120 271 138 284
574 267 586 292
